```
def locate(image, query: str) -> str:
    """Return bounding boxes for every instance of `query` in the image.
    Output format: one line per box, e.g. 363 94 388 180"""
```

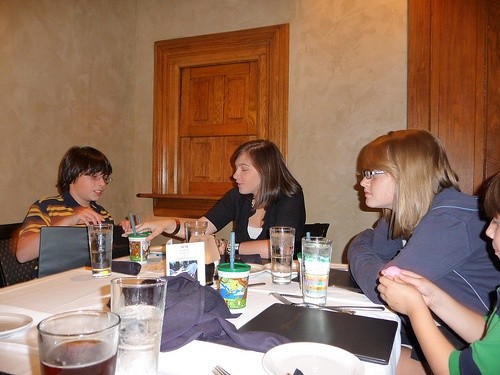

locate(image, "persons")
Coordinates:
121 140 306 258
378 173 500 375
347 129 500 375
16 146 131 280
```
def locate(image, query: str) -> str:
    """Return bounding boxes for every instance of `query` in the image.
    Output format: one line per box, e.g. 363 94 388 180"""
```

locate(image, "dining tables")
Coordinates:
0 245 403 375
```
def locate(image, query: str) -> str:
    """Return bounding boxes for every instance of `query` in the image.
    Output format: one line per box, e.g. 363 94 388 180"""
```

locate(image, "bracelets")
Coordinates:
170 218 180 235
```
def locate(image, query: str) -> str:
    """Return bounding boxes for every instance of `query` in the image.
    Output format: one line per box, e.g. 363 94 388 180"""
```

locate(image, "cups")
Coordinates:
302 237 332 306
138 233 151 256
216 263 251 309
37 310 121 375
110 278 167 375
298 253 330 291
128 235 148 264
88 224 113 277
270 227 296 284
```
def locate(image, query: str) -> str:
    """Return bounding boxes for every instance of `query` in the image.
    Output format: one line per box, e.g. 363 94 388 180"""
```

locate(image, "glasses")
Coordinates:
362 170 388 179
81 172 112 184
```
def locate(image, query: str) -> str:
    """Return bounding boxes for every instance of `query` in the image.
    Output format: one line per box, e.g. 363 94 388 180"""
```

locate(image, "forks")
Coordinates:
213 366 231 375
273 292 356 314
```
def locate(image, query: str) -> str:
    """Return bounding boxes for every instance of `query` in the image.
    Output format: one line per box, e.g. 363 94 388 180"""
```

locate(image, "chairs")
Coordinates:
297 223 332 261
0 239 40 287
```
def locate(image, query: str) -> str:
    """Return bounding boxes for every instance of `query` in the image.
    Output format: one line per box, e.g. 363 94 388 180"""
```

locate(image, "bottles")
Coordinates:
185 221 215 285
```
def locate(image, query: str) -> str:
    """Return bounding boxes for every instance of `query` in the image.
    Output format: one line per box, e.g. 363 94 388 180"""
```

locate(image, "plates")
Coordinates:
0 312 33 338
245 263 299 275
263 342 365 375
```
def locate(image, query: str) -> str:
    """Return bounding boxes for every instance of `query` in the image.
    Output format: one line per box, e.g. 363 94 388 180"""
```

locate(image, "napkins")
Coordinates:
220 253 262 264
86 258 141 275
157 272 289 353
